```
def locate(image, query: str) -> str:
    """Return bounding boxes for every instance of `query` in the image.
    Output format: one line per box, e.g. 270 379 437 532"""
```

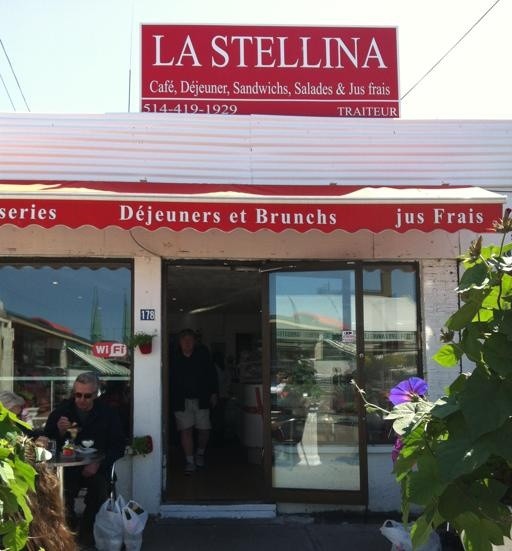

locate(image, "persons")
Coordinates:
0 390 26 424
329 368 395 443
273 368 329 444
170 328 218 477
45 375 128 551
211 352 232 450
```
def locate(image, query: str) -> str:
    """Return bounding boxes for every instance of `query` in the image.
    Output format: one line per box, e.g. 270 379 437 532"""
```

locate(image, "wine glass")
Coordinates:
81 440 94 453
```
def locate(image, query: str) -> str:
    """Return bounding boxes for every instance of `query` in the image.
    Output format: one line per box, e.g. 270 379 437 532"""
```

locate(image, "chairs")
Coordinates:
271 418 312 471
74 460 117 511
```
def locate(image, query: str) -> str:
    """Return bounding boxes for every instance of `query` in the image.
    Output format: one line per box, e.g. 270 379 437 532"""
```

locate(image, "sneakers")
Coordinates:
183 453 206 475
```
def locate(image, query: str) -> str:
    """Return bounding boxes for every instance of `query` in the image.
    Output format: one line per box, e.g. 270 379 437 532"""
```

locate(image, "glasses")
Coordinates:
75 391 96 398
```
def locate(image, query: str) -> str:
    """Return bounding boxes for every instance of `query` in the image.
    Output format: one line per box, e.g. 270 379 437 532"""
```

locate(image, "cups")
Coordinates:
46 440 56 460
